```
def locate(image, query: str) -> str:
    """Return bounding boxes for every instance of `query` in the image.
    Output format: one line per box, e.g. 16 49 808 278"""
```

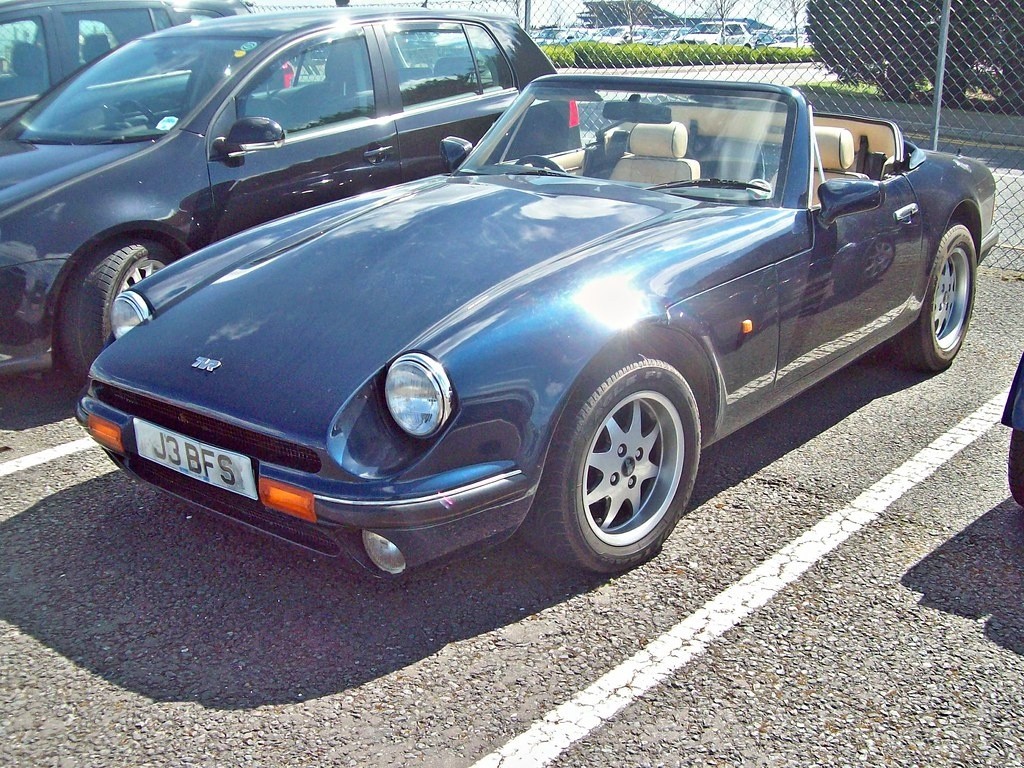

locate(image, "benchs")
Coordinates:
688 134 888 182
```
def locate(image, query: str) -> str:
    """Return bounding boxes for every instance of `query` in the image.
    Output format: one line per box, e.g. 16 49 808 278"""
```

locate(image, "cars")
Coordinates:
0 7 582 393
0 0 296 135
531 20 813 48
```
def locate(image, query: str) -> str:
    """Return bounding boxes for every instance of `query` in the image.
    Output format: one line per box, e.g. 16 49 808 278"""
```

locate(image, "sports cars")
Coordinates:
75 73 1000 578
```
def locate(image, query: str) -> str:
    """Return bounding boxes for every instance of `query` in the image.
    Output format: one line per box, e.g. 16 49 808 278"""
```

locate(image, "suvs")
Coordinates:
676 21 756 47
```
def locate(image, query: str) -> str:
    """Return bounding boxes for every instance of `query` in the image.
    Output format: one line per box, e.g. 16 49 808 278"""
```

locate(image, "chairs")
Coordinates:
318 44 375 116
609 121 700 188
432 56 472 96
246 72 288 124
771 126 870 205
